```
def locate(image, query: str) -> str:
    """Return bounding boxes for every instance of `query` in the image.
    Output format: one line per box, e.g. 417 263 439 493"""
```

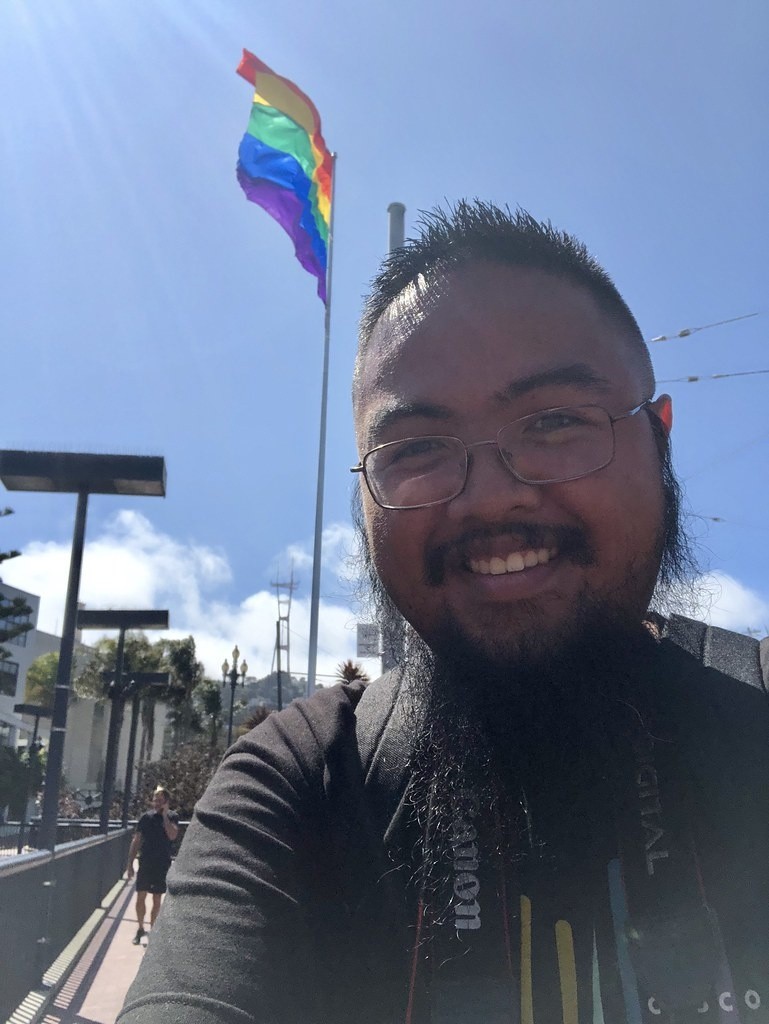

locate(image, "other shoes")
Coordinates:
133 929 145 944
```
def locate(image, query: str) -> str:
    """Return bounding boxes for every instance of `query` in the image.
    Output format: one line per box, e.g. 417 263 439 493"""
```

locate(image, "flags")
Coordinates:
234 48 334 308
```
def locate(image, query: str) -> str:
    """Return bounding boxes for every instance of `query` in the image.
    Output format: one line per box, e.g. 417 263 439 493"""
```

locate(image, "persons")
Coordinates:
115 197 769 1024
126 789 180 946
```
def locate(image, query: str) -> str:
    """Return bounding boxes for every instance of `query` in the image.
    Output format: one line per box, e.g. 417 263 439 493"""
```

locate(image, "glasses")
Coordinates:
350 397 651 512
